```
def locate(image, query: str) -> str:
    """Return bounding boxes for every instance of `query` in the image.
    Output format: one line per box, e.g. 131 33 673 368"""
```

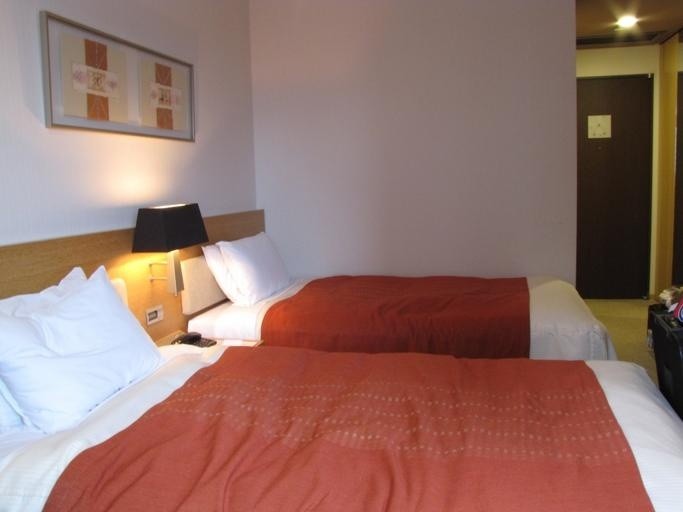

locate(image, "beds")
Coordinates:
0 343 683 511
189 274 619 361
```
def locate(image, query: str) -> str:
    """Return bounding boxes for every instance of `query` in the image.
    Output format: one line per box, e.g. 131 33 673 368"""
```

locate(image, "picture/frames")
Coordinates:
39 9 194 143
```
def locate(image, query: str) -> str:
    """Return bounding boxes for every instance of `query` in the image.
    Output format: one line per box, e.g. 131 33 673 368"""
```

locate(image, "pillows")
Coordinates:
0 265 166 435
218 231 291 305
0 266 92 437
201 244 243 304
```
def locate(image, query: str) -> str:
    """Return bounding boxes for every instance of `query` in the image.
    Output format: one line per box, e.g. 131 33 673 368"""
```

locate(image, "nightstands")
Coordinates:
158 330 265 348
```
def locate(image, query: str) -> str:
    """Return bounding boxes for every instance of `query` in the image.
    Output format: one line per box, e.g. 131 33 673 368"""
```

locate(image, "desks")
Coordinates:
648 304 682 423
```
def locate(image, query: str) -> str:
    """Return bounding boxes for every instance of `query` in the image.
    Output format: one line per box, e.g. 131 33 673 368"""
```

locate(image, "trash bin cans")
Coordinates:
646 303 669 352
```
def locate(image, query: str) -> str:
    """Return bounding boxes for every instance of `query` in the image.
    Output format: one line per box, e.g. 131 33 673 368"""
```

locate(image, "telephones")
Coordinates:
170 332 217 347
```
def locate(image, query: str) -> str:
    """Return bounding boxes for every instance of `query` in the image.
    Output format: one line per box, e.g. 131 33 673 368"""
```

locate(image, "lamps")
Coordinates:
132 202 208 293
